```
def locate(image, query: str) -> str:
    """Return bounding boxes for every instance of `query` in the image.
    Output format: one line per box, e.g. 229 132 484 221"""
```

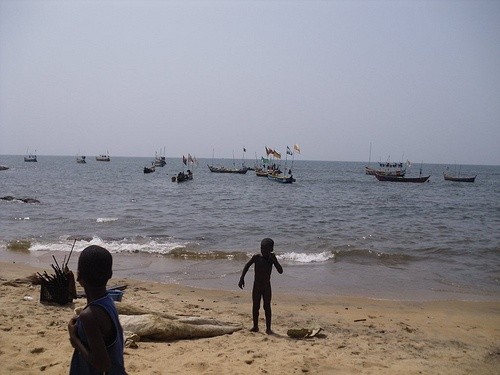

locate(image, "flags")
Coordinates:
183 156 188 165
265 144 300 159
188 153 193 162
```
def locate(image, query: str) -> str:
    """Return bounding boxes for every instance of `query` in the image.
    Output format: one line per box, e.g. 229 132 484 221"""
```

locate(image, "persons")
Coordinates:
238 238 283 335
67 245 128 375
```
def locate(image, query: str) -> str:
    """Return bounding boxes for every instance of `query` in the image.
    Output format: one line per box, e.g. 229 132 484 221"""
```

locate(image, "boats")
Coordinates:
442 171 477 182
152 160 167 168
95 156 111 162
267 171 297 184
76 158 86 164
365 166 407 177
0 165 9 170
206 164 248 174
143 165 157 174
255 169 283 177
241 163 264 171
23 156 38 163
374 171 432 183
177 171 194 183
